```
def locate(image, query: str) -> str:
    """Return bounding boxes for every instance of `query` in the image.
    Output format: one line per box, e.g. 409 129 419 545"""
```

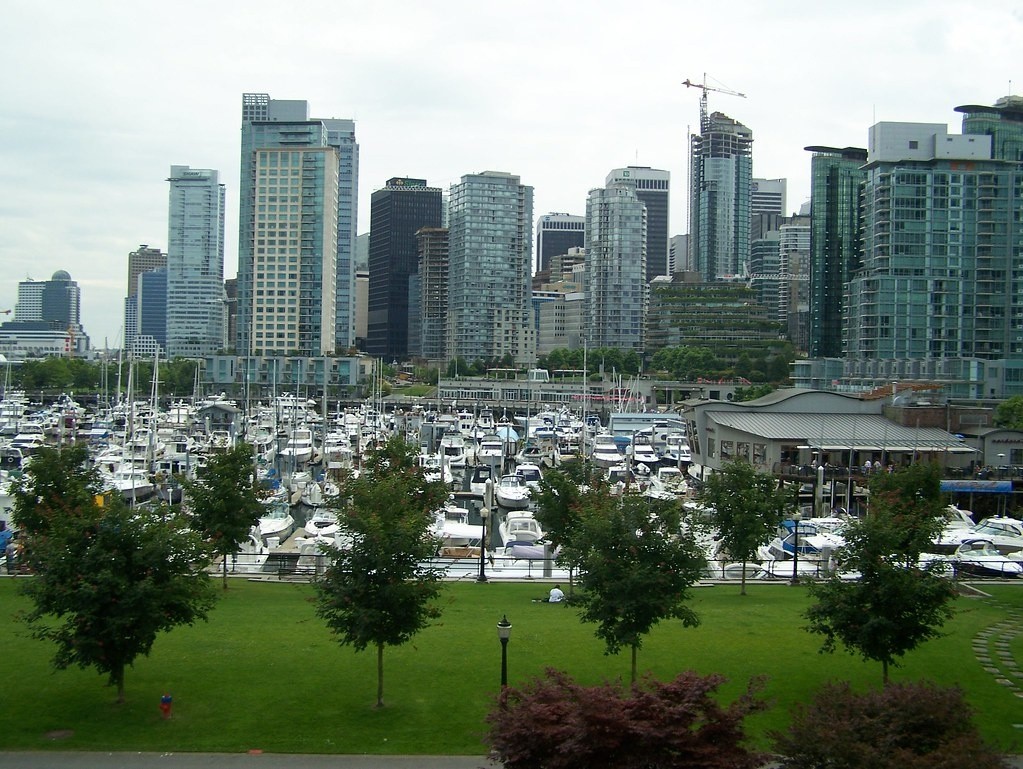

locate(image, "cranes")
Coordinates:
206 297 238 355
681 69 748 133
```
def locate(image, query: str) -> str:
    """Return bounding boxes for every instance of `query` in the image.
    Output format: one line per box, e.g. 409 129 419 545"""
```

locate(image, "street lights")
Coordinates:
495 614 513 757
474 506 490 586
997 453 1006 481
789 509 801 586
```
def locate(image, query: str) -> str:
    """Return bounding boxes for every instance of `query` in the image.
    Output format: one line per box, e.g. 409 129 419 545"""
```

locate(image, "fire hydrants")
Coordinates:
158 692 174 721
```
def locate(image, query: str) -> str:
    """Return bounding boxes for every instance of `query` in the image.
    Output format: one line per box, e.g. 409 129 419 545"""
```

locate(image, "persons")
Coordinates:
811 457 995 480
549 585 565 602
6 539 14 575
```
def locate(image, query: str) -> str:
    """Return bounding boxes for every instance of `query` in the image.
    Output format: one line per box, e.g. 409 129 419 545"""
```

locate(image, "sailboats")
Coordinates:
1 317 1023 583
